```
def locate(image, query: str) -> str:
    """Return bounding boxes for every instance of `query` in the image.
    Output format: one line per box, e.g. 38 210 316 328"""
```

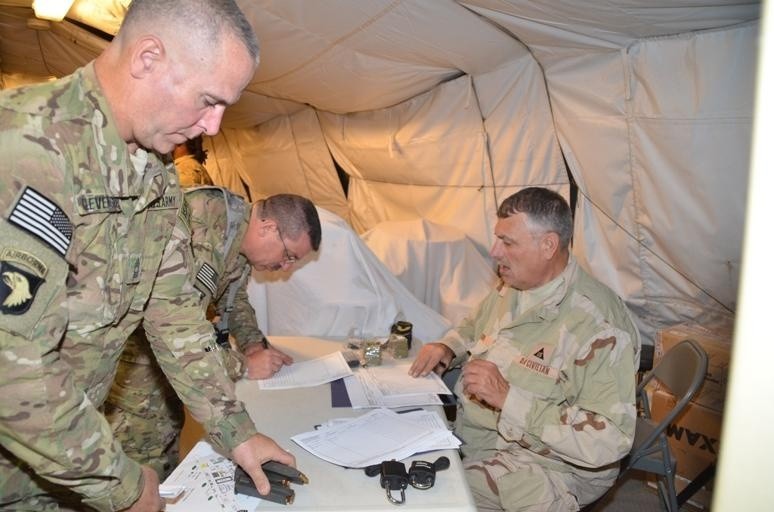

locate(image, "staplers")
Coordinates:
235 459 309 507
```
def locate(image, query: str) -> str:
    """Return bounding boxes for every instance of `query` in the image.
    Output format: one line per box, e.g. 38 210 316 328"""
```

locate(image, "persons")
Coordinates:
0 0 297 511
406 186 642 512
95 186 322 482
170 133 214 192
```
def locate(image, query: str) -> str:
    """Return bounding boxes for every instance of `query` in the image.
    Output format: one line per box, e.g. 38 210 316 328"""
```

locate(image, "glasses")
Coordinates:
278 228 297 265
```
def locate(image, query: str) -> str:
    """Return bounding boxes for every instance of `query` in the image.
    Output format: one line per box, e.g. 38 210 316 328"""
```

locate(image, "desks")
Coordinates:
229 332 477 512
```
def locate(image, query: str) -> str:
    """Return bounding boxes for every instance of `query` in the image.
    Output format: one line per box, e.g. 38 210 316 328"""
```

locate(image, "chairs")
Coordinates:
569 332 709 511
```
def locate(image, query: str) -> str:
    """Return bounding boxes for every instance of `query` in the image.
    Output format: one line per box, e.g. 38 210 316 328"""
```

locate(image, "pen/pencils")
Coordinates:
439 361 446 368
262 338 268 348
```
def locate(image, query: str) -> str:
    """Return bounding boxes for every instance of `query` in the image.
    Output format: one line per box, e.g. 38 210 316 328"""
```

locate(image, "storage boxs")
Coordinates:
638 321 735 512
